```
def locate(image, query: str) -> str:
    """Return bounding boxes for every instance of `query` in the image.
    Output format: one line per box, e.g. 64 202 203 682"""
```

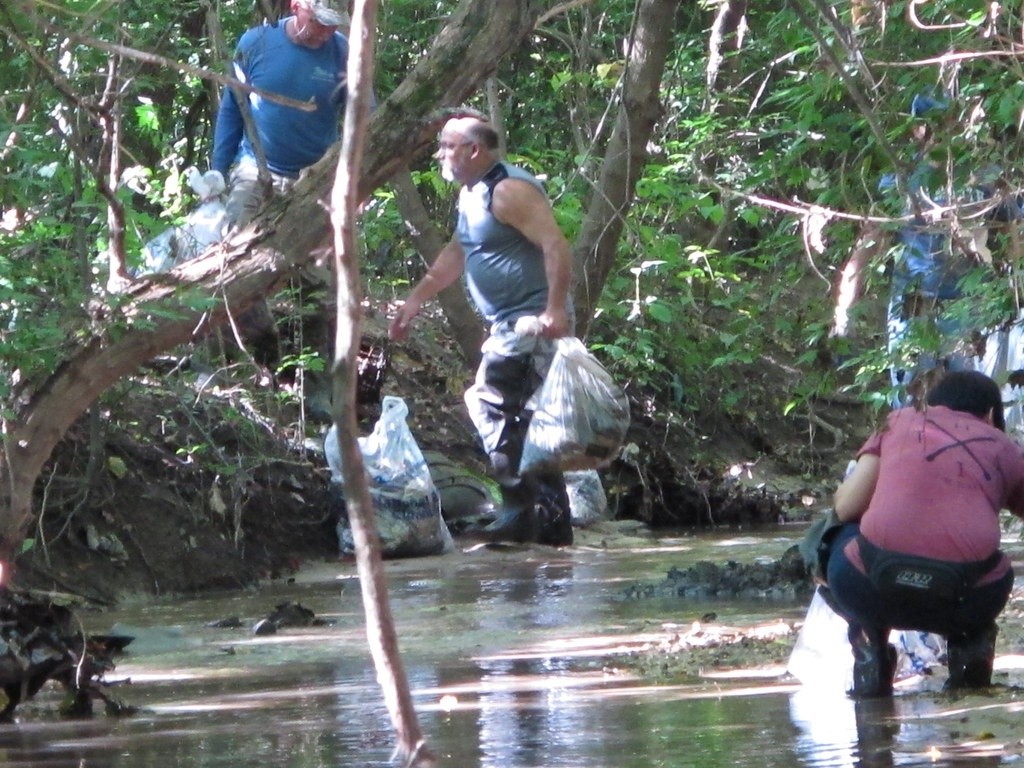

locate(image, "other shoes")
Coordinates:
258 369 289 387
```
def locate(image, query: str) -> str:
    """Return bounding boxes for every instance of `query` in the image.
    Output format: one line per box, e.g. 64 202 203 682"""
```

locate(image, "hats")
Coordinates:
300 0 353 26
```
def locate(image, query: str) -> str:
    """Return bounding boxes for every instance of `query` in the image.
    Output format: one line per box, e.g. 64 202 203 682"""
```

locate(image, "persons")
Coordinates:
833 89 1024 411
816 371 1024 699
198 0 381 425
385 107 572 549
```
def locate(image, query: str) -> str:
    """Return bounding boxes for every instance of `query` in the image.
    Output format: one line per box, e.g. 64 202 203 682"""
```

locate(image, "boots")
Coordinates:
941 630 1001 693
526 468 573 548
845 621 898 698
464 385 536 545
